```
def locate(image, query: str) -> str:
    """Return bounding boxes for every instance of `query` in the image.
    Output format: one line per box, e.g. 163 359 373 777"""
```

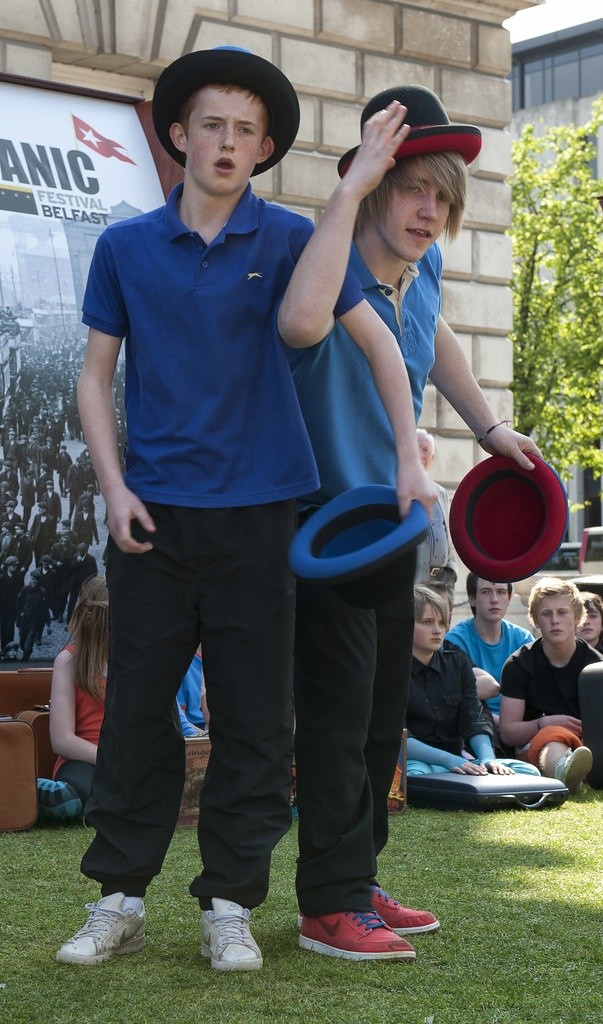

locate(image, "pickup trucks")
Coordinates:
511 525 603 608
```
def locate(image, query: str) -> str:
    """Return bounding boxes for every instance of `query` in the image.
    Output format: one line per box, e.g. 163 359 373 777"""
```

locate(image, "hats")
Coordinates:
152 47 302 176
289 485 427 608
449 451 567 582
336 86 483 178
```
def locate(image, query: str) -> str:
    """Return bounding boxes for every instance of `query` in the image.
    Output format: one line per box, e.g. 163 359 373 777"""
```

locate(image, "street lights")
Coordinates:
47 228 66 331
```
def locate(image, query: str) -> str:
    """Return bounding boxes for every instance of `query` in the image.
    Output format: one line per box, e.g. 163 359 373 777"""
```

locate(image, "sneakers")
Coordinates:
297 885 440 935
299 909 417 959
56 892 146 965
199 897 262 971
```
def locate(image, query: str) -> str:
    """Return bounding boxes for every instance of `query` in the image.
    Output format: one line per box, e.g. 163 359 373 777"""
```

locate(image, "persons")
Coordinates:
398 583 542 775
38 575 110 827
415 428 456 633
0 305 125 664
444 571 536 759
175 651 210 738
575 592 603 656
500 578 603 791
55 44 438 971
278 85 543 958
427 581 501 731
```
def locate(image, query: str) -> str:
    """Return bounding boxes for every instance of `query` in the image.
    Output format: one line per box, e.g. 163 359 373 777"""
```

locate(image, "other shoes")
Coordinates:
555 746 592 794
